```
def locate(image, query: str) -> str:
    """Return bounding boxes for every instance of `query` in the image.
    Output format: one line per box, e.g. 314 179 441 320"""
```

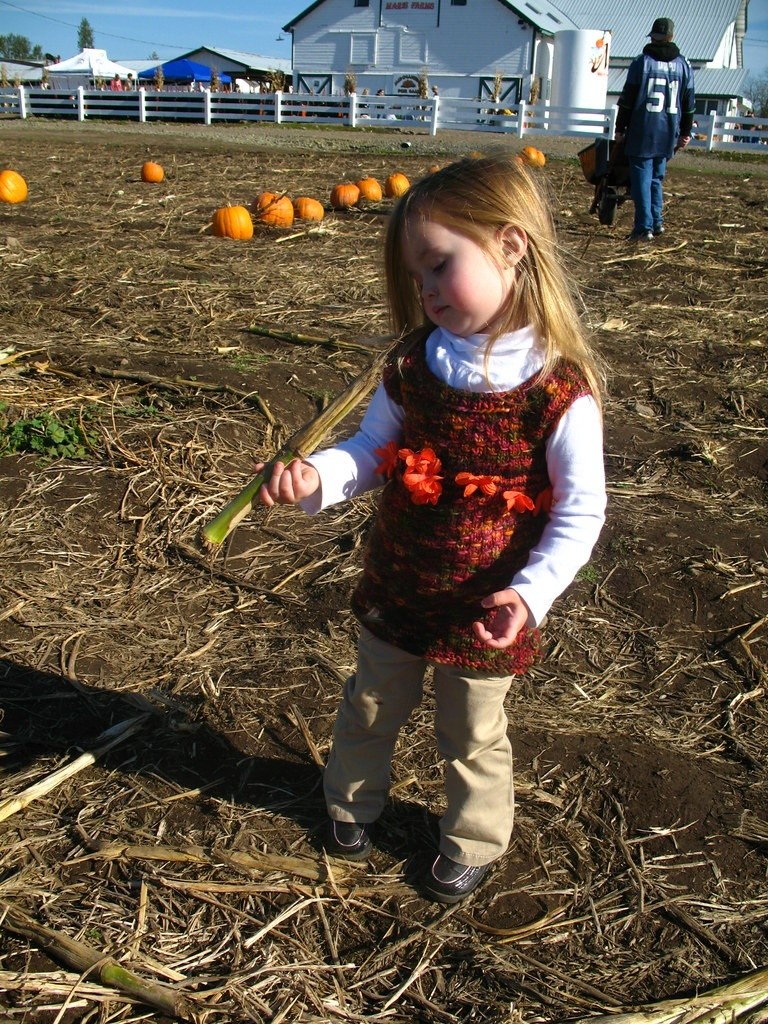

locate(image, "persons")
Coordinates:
614 18 695 239
360 88 387 120
14 78 23 90
261 154 609 900
733 109 767 151
40 76 51 91
431 84 441 119
110 73 133 92
186 80 230 93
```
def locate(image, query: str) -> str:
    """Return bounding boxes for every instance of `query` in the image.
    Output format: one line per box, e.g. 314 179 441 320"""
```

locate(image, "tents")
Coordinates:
138 57 233 94
41 48 138 91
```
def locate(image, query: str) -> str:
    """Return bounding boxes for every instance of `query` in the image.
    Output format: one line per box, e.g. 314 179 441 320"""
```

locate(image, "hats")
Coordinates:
646 18 674 41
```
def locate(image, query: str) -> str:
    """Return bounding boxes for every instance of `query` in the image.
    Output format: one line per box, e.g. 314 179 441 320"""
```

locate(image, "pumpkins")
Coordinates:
386 174 411 197
254 193 294 226
357 180 382 201
141 162 163 183
293 197 324 222
515 147 545 167
330 184 360 207
0 169 27 203
213 206 253 239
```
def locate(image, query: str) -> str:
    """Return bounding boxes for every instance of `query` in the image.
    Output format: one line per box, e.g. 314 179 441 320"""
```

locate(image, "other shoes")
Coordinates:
653 226 665 237
329 819 377 861
626 226 654 241
423 852 493 904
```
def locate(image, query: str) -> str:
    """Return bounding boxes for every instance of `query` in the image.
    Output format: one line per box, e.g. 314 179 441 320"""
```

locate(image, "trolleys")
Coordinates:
577 131 685 225
